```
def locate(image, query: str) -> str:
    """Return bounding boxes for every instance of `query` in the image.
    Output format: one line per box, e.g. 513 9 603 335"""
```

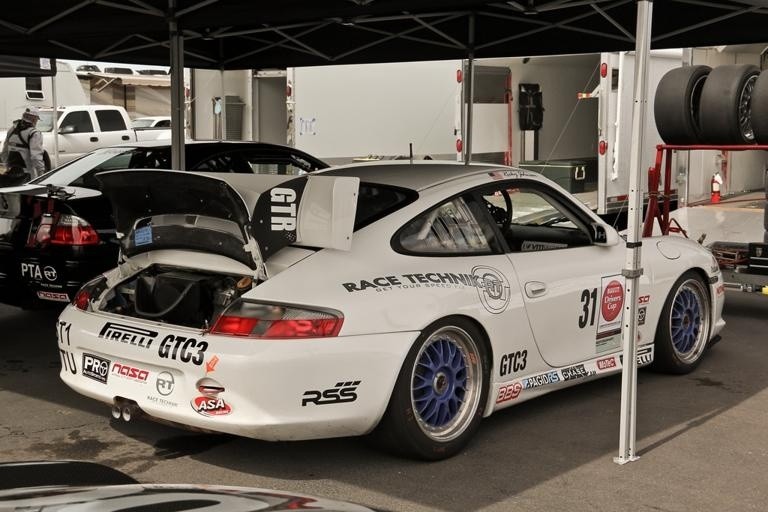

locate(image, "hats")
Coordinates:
25 106 40 120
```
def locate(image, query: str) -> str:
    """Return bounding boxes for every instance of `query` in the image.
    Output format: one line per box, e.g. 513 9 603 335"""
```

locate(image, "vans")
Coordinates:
0 103 171 180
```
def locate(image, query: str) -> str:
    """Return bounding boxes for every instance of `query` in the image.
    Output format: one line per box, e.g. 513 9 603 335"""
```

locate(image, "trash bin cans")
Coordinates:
212 95 246 141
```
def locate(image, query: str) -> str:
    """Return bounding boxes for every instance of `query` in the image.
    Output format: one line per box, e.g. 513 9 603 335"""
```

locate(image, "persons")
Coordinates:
4 108 45 177
0 151 31 185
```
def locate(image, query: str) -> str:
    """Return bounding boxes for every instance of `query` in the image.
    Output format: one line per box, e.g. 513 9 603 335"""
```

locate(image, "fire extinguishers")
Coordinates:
711 171 721 203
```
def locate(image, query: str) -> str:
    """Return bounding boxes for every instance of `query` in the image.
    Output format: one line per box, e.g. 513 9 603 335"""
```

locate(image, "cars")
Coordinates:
131 115 171 129
0 140 333 319
56 157 726 462
